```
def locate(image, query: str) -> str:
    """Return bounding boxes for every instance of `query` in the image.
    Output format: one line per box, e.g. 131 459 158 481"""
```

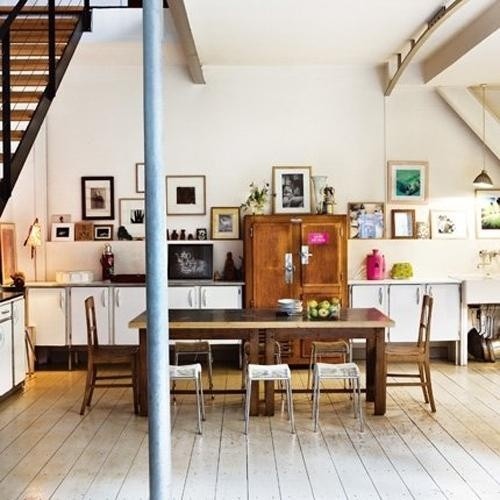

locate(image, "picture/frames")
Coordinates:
270 165 314 216
0 222 19 285
48 161 241 281
347 159 500 240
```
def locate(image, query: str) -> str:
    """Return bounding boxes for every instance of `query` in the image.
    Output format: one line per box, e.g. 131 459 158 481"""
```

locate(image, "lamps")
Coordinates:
24 215 43 281
471 84 494 187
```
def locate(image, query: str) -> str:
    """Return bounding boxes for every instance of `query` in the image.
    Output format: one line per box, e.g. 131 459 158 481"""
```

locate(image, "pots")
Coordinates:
467 308 500 363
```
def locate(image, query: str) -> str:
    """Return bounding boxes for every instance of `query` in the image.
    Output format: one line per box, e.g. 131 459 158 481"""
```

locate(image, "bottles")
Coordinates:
224 251 237 281
166 228 185 241
101 243 114 281
364 248 385 281
322 190 335 214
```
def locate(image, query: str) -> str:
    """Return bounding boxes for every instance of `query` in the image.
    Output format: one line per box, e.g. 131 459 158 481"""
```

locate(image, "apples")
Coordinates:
309 296 341 319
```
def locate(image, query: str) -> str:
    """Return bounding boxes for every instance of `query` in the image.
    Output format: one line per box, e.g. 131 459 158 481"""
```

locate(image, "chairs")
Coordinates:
80 295 141 418
382 295 437 413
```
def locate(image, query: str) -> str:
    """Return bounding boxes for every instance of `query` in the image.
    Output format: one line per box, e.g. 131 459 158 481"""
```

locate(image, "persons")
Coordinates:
130 210 144 223
283 175 304 208
91 190 104 208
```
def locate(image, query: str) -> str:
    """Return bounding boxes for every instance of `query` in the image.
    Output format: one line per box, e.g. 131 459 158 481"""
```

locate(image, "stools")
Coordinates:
168 337 367 435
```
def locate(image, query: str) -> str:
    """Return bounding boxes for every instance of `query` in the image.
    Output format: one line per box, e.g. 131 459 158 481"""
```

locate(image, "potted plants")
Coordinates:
240 178 270 217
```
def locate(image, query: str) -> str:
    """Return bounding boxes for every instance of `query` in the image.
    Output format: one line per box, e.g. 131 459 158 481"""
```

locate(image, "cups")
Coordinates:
310 174 328 214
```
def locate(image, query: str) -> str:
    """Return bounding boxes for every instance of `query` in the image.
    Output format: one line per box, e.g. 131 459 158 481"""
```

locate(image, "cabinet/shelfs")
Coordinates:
25 287 72 371
243 213 350 370
386 283 462 344
69 287 147 370
169 285 242 367
8 296 26 395
351 285 386 345
0 291 14 401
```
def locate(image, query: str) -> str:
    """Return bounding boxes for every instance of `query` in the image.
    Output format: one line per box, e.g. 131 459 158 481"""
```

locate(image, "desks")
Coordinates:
127 305 397 416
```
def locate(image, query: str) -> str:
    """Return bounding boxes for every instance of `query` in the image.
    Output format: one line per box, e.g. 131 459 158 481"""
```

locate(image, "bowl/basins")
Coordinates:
276 297 304 316
